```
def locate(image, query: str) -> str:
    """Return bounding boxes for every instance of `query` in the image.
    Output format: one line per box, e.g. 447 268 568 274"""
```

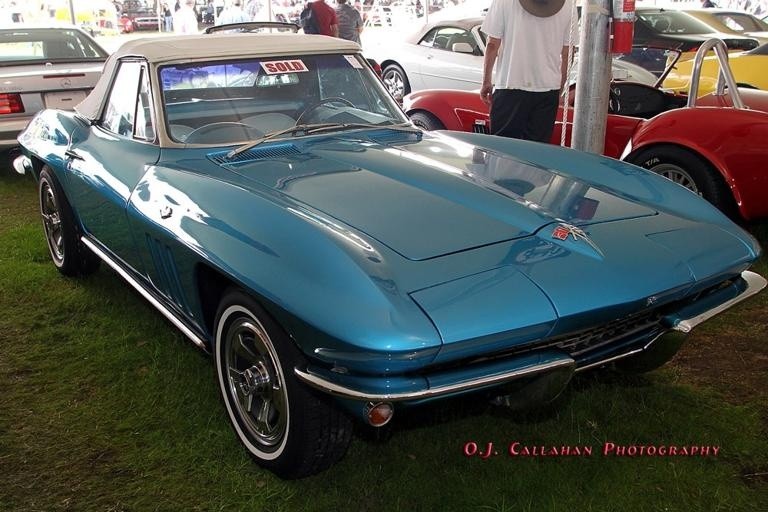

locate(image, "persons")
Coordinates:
300 0 363 104
466 146 553 199
479 1 579 143
162 1 265 35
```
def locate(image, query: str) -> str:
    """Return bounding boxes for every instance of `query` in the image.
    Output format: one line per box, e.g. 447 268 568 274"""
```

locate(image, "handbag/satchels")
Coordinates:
300 2 320 34
